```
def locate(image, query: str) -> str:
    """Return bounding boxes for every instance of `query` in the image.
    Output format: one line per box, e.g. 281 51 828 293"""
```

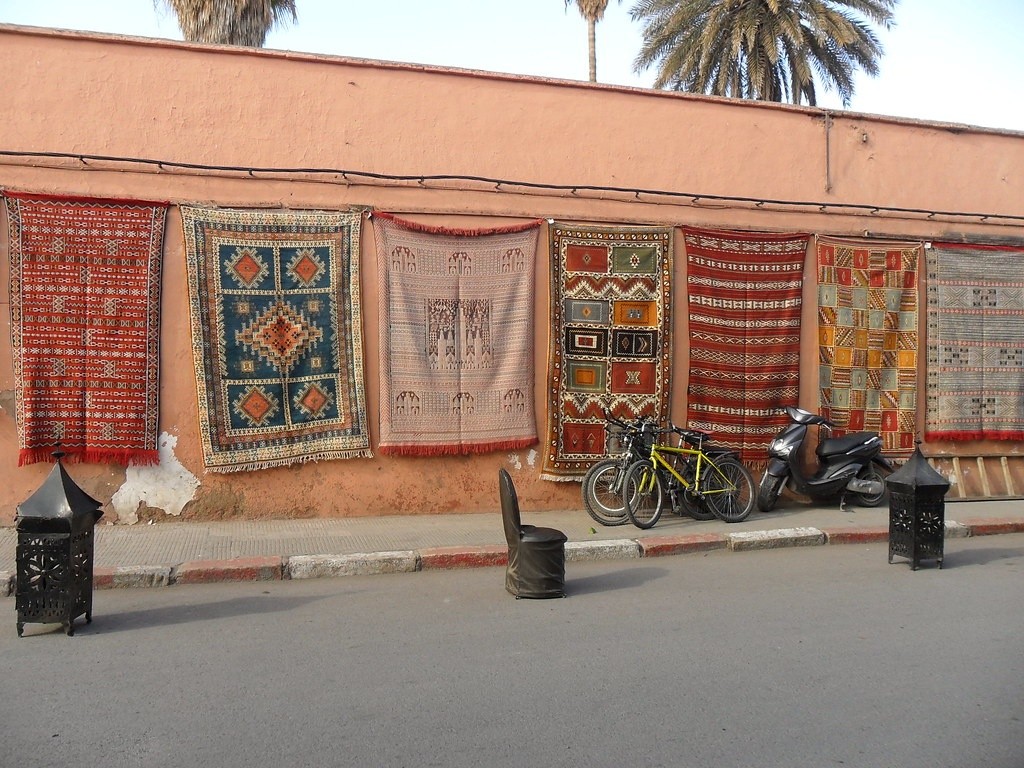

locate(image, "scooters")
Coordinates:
756 402 892 512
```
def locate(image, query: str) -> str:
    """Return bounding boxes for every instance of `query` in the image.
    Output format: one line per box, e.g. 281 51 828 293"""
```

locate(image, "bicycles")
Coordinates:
580 405 757 533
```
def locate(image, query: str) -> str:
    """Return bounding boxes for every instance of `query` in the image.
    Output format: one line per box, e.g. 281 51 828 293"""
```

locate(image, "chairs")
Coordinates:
498 468 567 600
